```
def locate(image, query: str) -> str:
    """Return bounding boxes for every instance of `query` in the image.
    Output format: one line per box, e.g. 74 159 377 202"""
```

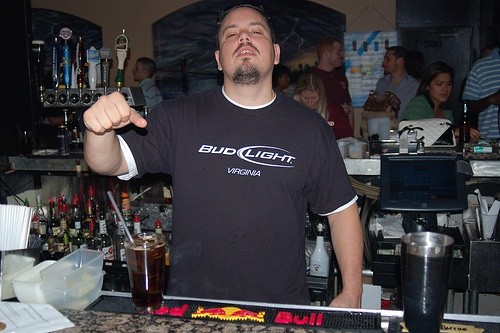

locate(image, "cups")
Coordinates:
401 232 453 333
123 232 169 311
476 204 500 240
17 130 32 152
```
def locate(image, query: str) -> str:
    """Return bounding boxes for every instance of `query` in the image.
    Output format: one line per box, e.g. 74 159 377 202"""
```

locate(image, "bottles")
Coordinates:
23 186 163 266
371 42 381 93
361 41 371 94
382 39 389 78
458 100 470 151
350 40 362 94
304 210 332 277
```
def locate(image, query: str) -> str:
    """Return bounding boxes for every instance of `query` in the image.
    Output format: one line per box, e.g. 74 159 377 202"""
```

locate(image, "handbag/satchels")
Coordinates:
364 90 401 111
398 118 457 148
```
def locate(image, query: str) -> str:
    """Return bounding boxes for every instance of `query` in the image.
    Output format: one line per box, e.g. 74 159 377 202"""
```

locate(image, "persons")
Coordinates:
463 45 500 141
83 0 364 309
271 66 291 96
402 61 480 142
376 46 420 123
134 57 163 204
308 36 353 140
291 72 330 243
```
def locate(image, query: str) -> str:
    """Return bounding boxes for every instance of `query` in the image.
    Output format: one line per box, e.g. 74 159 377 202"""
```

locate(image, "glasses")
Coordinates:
216 1 273 28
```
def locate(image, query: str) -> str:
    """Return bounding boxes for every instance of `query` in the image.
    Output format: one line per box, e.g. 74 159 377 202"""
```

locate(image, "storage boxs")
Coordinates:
0 248 105 311
462 222 500 291
